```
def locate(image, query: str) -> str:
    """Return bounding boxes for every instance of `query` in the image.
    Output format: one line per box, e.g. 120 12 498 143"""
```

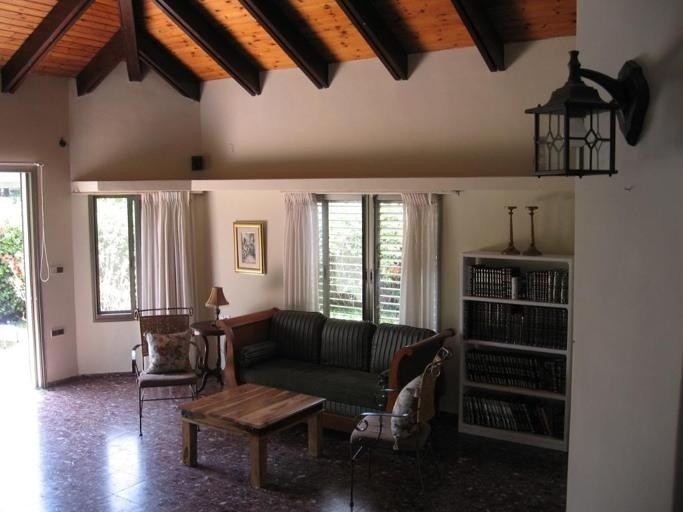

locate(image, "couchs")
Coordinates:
215 307 455 435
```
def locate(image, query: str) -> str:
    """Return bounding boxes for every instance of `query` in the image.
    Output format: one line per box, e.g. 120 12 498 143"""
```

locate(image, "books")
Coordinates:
462 263 568 441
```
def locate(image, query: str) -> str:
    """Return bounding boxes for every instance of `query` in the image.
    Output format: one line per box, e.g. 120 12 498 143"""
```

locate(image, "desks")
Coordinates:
189 320 227 393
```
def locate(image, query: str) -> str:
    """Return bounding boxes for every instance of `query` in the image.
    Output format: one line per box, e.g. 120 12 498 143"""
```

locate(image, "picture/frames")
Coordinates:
232 222 265 275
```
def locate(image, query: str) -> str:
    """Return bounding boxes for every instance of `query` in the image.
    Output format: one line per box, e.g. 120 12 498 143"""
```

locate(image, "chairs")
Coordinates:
129 306 203 438
347 345 453 511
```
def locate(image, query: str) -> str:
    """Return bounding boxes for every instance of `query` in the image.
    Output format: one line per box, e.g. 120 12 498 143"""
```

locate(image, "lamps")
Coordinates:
522 49 650 178
205 286 228 326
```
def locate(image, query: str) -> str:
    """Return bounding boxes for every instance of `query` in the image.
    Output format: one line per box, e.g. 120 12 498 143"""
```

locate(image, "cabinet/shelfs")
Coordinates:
455 250 573 454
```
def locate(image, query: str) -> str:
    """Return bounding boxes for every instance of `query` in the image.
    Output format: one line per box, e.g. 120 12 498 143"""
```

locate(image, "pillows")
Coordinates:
389 373 422 451
139 328 192 374
